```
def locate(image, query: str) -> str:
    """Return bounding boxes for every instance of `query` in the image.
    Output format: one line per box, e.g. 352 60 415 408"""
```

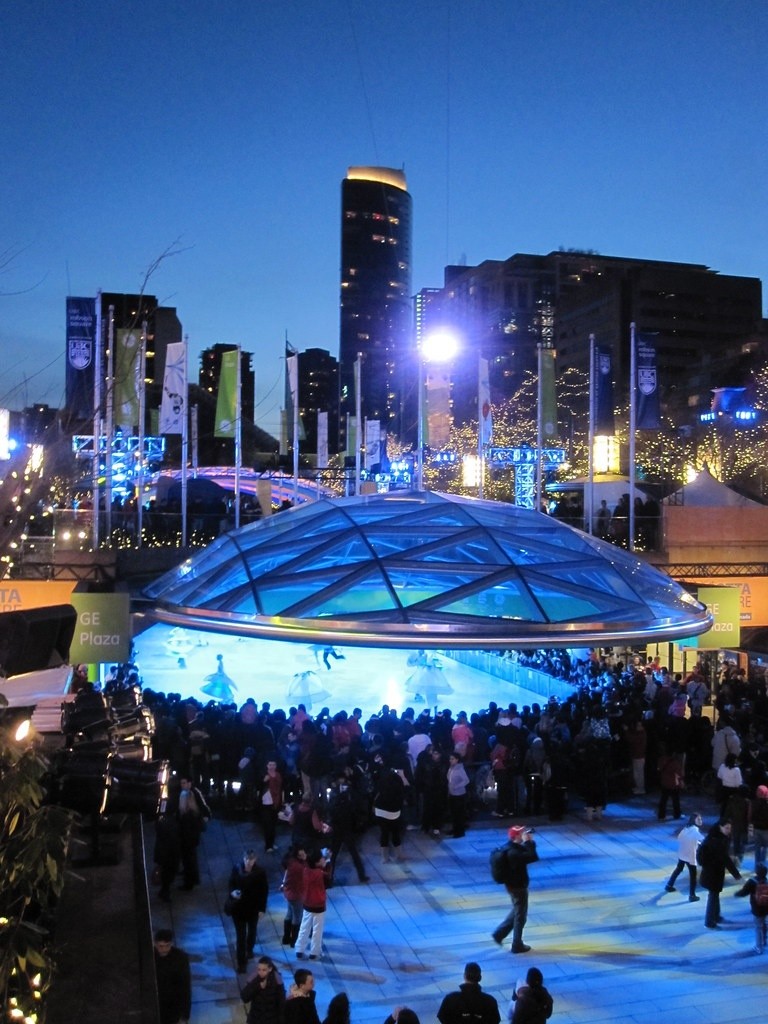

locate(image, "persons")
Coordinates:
28 494 664 553
0 649 768 1024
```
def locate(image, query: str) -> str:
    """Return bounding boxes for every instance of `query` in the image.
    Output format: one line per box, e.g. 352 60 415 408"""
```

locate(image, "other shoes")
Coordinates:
689 896 700 902
665 887 676 892
753 946 763 954
511 944 530 953
582 807 602 820
706 923 722 931
493 933 503 944
632 788 646 793
492 811 514 819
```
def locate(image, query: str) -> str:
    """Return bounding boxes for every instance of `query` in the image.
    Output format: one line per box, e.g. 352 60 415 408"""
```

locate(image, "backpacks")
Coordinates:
749 876 768 904
490 846 515 884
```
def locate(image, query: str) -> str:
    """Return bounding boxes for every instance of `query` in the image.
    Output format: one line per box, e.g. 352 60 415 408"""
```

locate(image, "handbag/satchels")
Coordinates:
688 699 692 708
224 896 240 917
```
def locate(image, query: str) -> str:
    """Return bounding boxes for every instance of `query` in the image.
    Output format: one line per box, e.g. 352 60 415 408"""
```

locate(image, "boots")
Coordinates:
354 860 370 882
393 845 413 860
290 925 300 948
380 846 389 863
282 920 292 944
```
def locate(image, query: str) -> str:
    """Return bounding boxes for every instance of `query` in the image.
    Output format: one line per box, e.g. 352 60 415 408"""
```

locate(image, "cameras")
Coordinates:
525 827 535 835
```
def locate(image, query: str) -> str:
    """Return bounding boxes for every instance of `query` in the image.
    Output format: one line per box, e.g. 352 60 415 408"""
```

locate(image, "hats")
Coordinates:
507 825 526 841
756 785 768 800
